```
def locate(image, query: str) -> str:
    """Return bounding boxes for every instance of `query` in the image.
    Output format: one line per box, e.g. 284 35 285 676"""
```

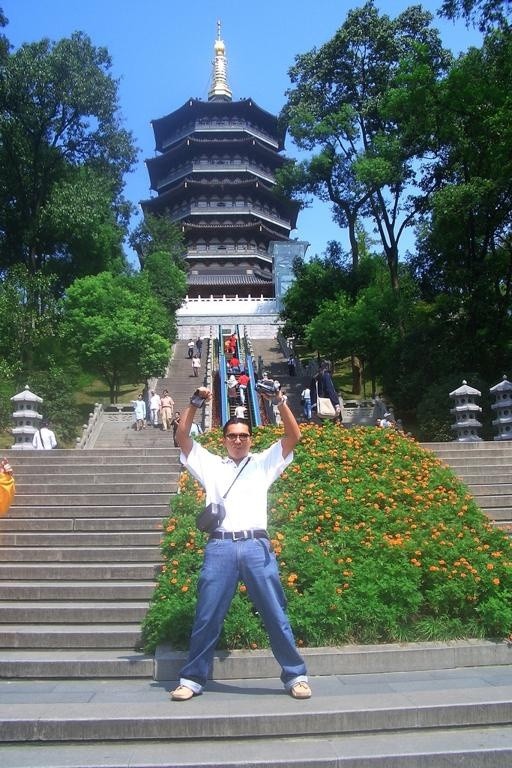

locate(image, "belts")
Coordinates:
209 529 270 541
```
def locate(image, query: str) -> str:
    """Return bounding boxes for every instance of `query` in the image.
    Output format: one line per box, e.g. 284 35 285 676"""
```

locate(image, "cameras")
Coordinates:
255 379 281 396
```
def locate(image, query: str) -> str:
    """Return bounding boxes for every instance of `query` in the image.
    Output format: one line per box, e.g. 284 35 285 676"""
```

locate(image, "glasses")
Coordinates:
226 433 249 440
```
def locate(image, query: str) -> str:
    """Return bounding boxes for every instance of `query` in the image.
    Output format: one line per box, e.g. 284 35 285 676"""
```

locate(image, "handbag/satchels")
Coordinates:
316 397 336 419
195 502 226 533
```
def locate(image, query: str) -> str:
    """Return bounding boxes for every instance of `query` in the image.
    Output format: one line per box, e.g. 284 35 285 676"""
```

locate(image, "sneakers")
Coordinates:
172 684 193 700
292 679 313 699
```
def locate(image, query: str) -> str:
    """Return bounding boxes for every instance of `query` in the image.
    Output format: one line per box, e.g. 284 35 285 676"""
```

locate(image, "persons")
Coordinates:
172 379 313 701
33 420 57 450
131 332 394 447
0 457 16 517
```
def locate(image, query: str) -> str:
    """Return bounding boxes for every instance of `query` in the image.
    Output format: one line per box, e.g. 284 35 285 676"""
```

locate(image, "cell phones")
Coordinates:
190 389 206 408
